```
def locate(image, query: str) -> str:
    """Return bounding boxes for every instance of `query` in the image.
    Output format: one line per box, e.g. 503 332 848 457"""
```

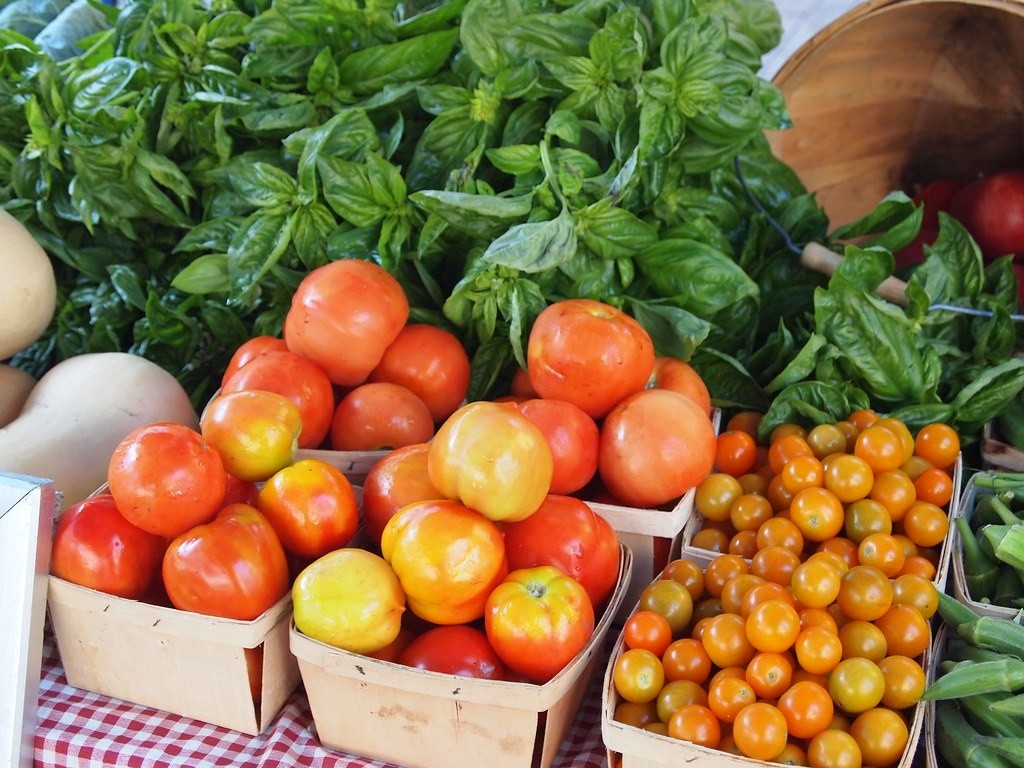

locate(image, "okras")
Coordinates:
921 474 1024 768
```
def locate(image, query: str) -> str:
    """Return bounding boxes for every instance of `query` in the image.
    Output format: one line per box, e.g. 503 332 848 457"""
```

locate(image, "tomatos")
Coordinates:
52 260 959 766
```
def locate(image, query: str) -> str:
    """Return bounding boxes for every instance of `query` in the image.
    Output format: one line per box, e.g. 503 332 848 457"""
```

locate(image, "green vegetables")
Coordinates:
0 0 1024 460
